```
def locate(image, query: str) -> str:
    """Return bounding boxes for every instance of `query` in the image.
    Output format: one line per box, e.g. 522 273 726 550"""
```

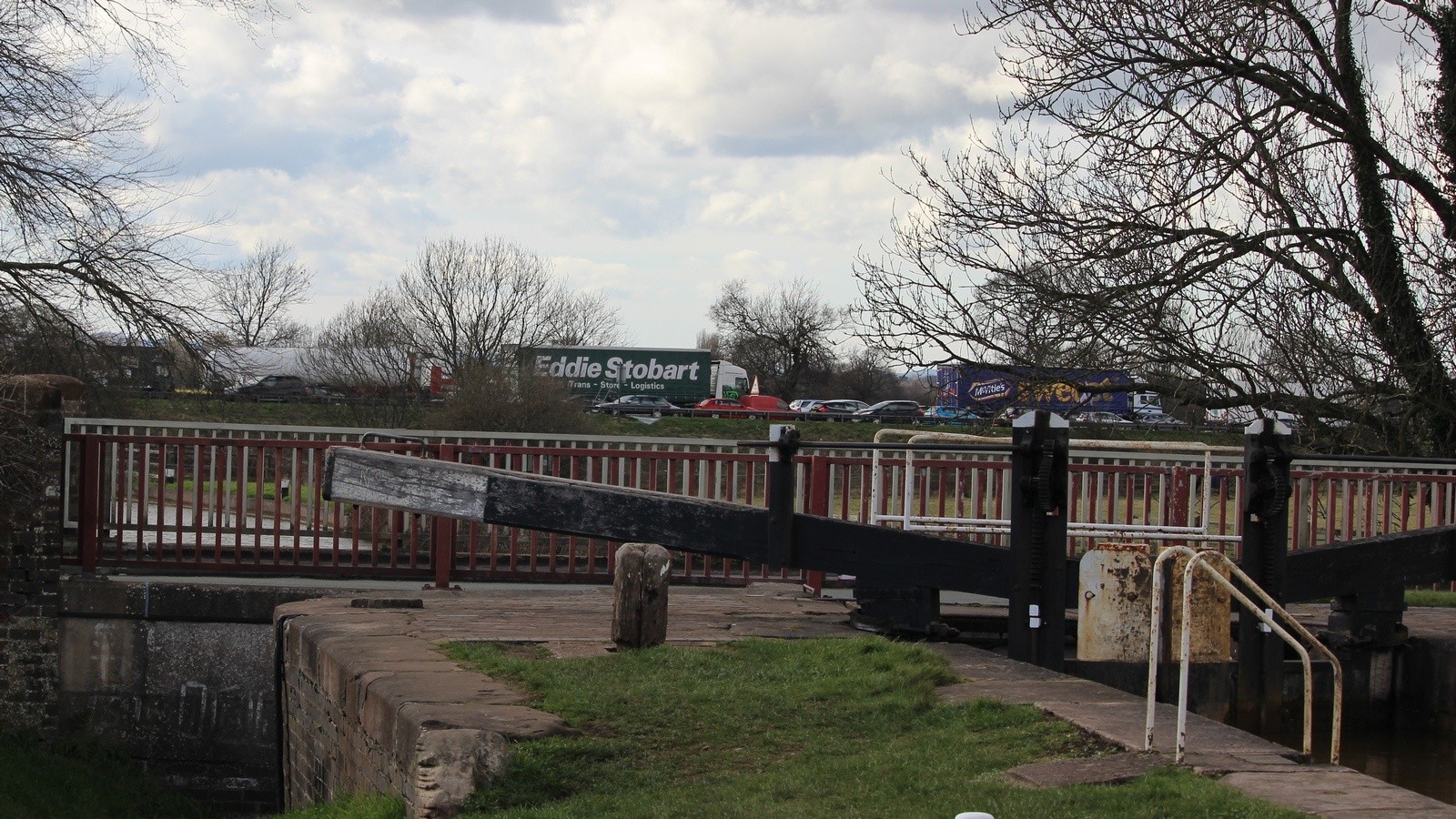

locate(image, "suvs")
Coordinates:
1075 411 1133 427
809 402 864 423
789 399 826 413
850 400 927 426
740 395 798 412
825 400 871 409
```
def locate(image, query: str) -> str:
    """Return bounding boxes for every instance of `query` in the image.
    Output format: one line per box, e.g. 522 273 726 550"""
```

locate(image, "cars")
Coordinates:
560 394 614 414
692 398 761 419
919 406 982 427
1132 413 1184 425
1277 418 1303 429
590 394 683 419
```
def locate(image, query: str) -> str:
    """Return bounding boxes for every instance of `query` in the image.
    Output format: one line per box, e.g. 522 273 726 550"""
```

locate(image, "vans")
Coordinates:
997 407 1032 420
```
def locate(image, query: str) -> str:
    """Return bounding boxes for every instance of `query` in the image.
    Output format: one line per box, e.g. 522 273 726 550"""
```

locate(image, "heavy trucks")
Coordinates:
202 346 462 405
500 343 751 414
935 361 1163 418
